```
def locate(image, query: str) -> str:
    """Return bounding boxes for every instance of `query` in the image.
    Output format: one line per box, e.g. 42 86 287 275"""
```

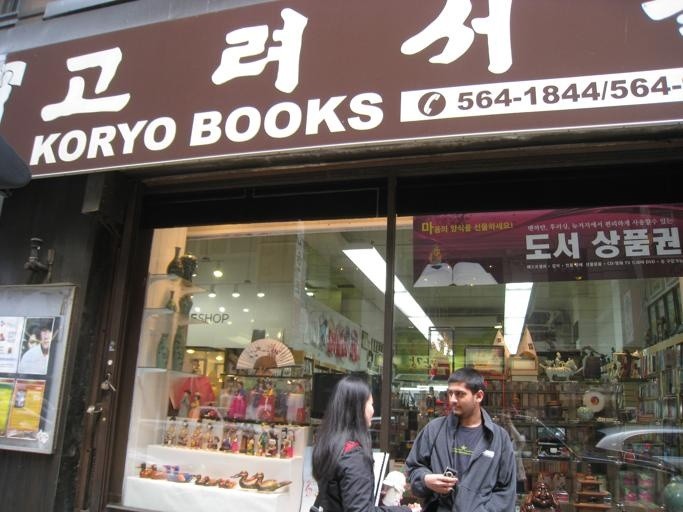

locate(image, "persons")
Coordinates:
139 377 306 492
19 320 52 374
308 366 516 512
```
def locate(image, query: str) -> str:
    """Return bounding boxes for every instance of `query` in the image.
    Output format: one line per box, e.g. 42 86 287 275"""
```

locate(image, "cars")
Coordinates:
491 412 683 512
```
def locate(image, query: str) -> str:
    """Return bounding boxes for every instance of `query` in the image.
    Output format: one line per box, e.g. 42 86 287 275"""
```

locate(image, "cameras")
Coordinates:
443 466 457 478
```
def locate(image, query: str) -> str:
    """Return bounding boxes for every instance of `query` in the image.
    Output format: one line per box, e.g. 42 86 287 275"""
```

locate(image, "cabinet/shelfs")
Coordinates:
117 407 310 512
408 334 682 478
